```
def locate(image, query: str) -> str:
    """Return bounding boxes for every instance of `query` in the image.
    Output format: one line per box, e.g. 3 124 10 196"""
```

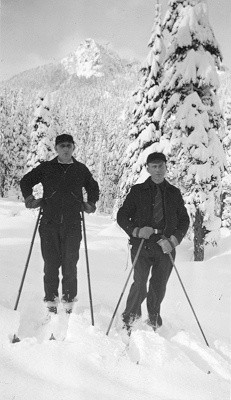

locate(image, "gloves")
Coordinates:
25 194 43 208
82 201 96 213
132 226 155 239
156 235 180 254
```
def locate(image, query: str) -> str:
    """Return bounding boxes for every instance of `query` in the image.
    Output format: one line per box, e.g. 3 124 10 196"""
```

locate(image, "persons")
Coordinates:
20 134 99 322
116 152 190 338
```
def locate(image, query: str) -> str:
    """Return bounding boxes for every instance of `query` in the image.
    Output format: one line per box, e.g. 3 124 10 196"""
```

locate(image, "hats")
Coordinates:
56 134 74 145
147 152 167 164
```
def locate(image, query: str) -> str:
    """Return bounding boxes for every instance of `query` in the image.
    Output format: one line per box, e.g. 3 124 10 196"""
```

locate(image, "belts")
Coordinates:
154 229 162 234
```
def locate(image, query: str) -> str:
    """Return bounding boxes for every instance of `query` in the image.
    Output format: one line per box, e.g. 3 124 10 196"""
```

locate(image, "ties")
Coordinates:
153 185 164 225
